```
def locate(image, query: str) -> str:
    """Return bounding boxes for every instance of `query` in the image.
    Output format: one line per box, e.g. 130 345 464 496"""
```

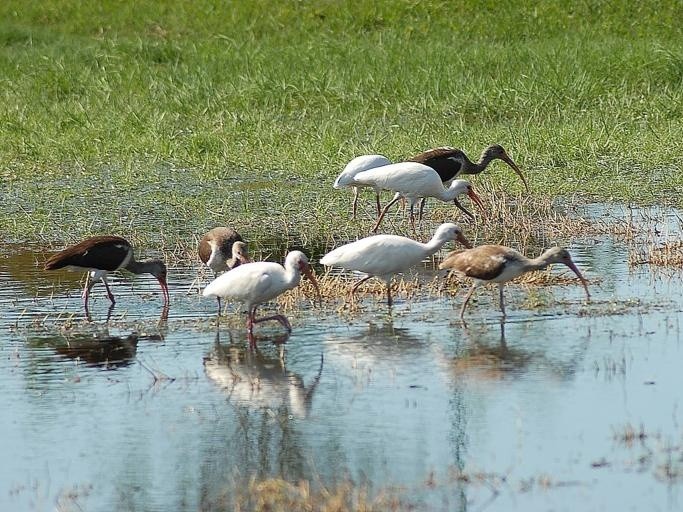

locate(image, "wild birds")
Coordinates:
403 142 531 224
330 153 406 219
201 249 321 334
318 222 471 300
437 244 589 321
352 160 486 233
203 334 325 419
197 225 252 313
41 233 171 318
